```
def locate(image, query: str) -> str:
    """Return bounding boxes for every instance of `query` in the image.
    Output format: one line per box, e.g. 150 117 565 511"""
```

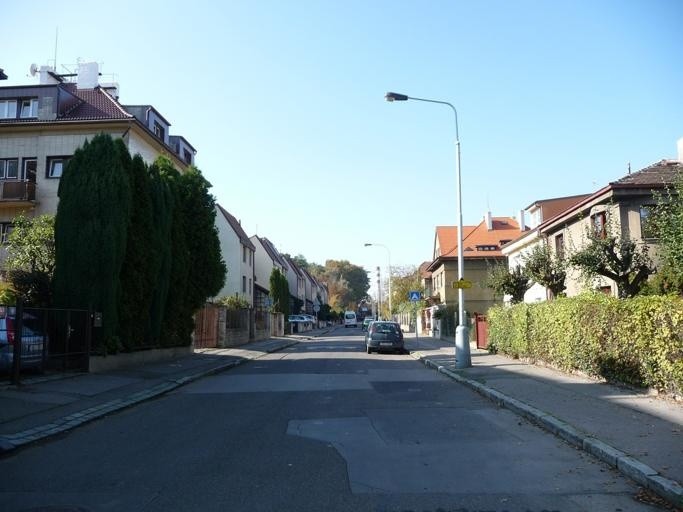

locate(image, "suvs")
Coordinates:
365 320 404 356
0 313 52 372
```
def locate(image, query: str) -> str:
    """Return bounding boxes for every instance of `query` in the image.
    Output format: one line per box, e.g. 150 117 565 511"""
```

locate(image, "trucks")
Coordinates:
343 309 356 329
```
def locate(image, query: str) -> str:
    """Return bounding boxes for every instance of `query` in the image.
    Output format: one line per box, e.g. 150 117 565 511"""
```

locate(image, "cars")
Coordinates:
287 314 333 329
362 318 374 332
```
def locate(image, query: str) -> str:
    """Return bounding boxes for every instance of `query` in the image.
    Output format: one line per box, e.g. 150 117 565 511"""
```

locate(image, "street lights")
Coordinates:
363 241 393 322
384 92 473 371
365 268 390 318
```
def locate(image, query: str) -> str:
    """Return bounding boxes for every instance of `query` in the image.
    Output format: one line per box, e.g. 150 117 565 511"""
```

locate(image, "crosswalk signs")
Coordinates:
409 290 420 301
